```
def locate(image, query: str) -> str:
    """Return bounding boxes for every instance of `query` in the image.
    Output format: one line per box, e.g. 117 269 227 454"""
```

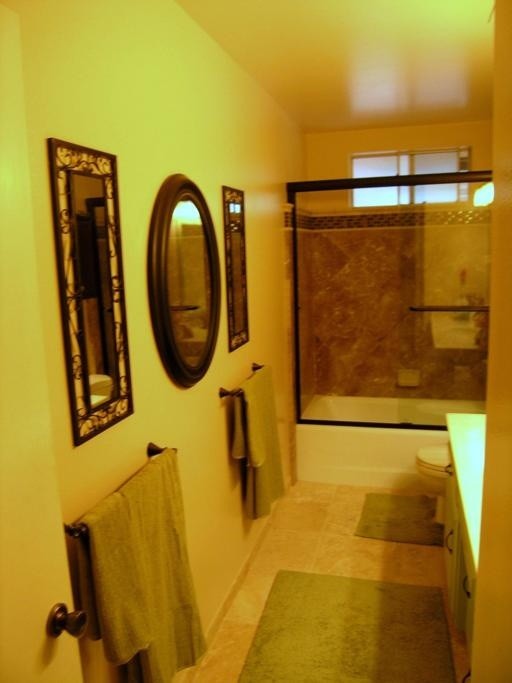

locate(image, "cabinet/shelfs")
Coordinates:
440 439 475 660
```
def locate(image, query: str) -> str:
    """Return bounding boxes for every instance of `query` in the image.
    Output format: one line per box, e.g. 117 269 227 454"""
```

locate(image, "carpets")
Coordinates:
238 568 458 683
352 491 444 548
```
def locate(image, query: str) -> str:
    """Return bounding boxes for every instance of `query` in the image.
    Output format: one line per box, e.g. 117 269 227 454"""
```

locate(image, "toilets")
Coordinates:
416 443 451 497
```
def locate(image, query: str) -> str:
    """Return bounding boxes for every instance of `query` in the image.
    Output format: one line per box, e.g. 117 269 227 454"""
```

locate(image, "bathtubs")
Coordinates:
296 394 486 492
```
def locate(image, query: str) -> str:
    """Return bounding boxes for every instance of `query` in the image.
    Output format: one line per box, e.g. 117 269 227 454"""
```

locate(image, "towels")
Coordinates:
115 449 207 682
73 489 154 669
249 365 287 522
231 379 272 468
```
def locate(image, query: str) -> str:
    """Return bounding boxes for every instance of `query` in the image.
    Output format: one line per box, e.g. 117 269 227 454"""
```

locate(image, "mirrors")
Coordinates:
147 172 222 388
220 184 250 353
47 135 135 449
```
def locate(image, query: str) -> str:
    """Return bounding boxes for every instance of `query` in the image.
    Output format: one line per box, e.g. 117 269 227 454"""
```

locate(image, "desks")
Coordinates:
421 308 489 399
